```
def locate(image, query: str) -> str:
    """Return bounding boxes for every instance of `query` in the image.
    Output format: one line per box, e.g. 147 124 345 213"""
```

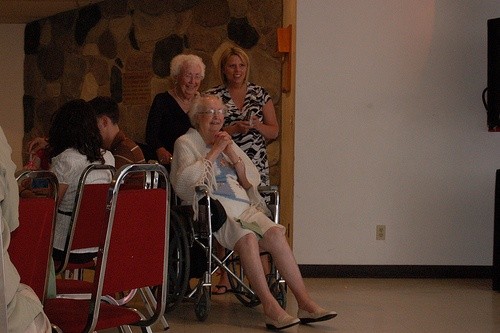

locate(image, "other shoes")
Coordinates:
186 282 206 299
297 307 337 324
265 312 300 331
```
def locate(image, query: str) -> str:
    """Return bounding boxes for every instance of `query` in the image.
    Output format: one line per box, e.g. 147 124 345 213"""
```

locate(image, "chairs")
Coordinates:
8 170 59 306
40 163 171 333
54 164 116 280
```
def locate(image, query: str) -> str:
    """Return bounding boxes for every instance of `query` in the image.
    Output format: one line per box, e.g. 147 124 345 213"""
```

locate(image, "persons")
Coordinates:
87 96 147 183
0 126 52 333
143 54 209 300
200 43 280 297
168 95 338 331
18 99 116 266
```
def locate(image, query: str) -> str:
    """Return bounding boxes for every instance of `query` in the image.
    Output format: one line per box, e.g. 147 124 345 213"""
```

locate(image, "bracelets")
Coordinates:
233 157 242 166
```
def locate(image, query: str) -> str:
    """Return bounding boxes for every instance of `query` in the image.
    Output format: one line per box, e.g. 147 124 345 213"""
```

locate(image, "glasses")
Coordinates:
195 108 226 117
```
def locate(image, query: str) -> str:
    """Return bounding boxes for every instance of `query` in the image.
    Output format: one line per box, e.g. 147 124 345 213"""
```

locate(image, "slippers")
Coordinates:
211 284 235 294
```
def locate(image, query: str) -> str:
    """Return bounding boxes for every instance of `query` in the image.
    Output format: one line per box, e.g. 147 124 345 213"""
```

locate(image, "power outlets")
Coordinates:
376 225 386 241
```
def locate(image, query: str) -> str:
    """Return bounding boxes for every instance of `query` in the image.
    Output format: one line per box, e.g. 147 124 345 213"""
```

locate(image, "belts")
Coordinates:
57 209 73 217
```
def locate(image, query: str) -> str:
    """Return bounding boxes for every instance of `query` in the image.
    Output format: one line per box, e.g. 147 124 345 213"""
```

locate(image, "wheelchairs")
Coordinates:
150 158 289 321
